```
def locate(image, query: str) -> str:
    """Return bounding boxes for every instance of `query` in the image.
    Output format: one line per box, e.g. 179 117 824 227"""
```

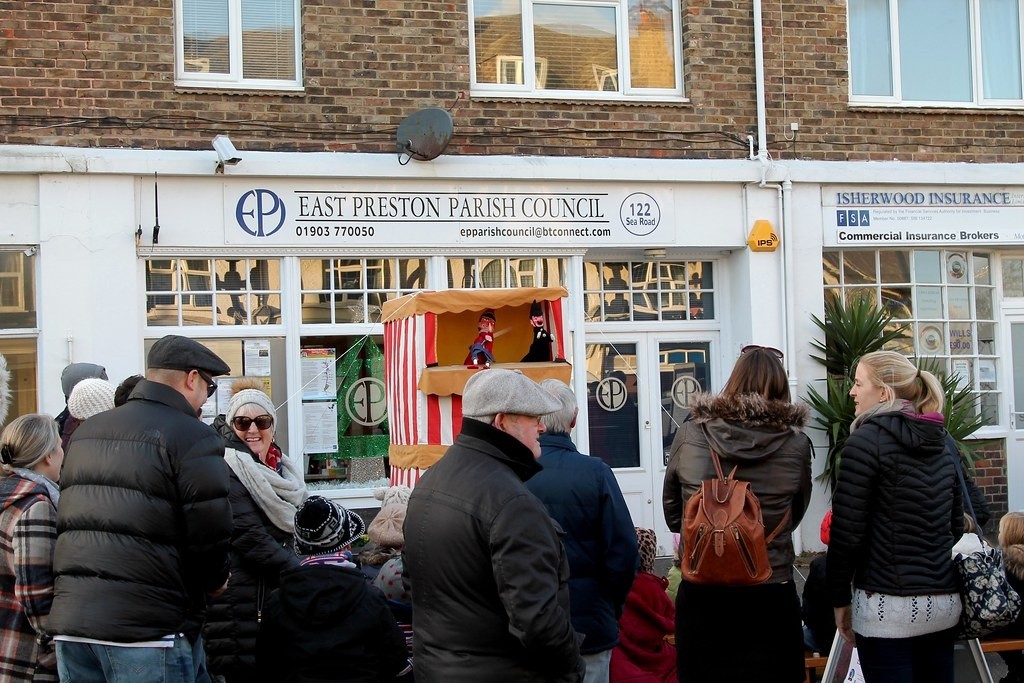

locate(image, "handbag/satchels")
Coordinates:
951 532 1021 640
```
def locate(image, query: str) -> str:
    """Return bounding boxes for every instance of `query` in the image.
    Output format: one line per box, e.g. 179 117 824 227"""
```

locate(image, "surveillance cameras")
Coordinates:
211 136 243 167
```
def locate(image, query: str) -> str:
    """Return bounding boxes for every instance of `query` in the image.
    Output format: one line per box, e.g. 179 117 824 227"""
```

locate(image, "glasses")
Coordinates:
490 413 542 425
198 371 217 397
741 345 784 366
232 415 272 431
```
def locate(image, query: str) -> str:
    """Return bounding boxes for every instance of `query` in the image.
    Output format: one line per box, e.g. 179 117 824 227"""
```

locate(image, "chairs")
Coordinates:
495 54 547 89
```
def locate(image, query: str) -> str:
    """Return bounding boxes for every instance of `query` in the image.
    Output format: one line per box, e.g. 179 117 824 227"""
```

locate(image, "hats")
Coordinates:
62 363 108 396
374 486 412 509
820 509 834 545
225 377 277 437
147 335 231 375
367 504 406 544
68 379 115 420
674 533 681 550
462 368 564 416
294 495 365 559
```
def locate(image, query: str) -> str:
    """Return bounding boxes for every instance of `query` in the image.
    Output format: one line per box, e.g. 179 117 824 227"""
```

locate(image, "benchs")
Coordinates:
805 635 1024 683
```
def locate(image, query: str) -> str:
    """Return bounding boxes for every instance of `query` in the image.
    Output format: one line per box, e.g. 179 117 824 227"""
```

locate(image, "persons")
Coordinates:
662 344 816 683
0 335 682 683
823 351 967 683
801 508 1024 683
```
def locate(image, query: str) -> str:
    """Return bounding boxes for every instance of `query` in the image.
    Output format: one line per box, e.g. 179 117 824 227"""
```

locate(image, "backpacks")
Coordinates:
681 447 791 585
